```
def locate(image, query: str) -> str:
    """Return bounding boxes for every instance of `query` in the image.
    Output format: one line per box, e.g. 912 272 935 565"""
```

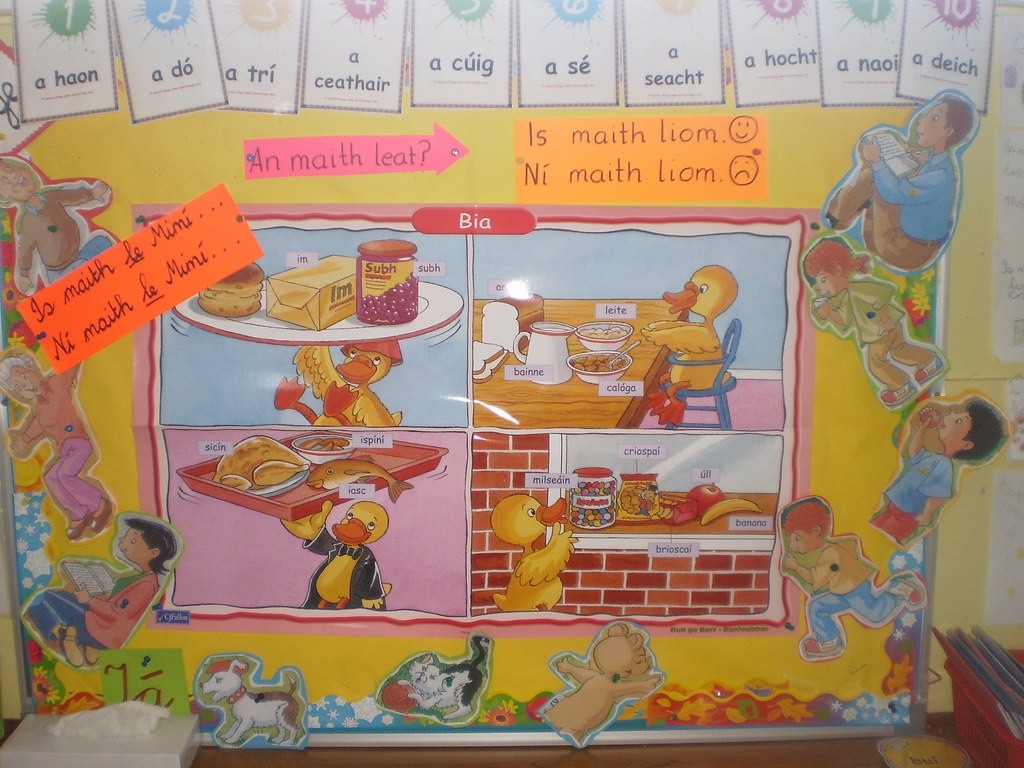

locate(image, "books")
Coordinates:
931 625 1024 740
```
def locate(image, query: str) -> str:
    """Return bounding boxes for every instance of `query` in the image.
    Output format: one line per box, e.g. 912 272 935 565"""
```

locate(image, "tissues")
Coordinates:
0 699 204 768
